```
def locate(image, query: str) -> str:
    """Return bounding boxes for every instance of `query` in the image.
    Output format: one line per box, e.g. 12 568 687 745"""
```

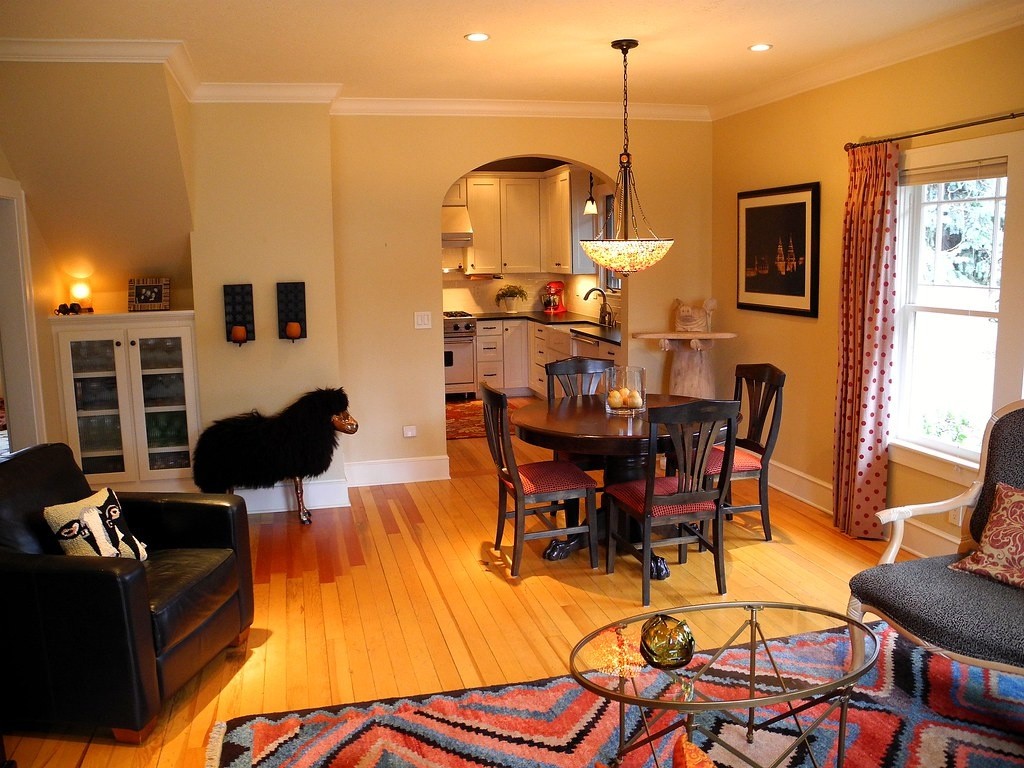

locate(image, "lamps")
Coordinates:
578 39 676 279
583 172 598 215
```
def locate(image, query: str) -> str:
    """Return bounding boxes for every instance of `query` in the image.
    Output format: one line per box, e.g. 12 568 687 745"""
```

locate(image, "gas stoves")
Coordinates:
443 311 477 336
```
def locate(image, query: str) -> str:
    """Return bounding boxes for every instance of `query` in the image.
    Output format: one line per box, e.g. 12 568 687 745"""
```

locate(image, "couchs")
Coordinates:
0 442 254 744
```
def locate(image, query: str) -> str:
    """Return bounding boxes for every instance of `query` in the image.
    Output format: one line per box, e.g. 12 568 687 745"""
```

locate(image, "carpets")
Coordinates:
445 395 521 440
204 619 1023 767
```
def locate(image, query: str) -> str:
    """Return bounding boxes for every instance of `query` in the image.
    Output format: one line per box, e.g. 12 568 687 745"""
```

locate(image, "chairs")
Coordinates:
843 400 1024 681
601 397 740 607
479 383 598 576
545 355 614 517
665 363 786 552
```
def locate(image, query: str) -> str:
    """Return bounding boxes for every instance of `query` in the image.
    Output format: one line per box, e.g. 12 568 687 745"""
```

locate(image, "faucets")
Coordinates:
583 287 607 326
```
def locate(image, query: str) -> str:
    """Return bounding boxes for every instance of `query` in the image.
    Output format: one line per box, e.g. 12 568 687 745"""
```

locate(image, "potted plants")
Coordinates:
495 285 528 313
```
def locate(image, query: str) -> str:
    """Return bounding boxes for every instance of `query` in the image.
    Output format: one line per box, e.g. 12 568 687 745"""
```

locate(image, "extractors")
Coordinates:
442 205 474 245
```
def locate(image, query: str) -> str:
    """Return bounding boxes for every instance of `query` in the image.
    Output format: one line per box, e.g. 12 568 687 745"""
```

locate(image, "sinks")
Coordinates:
547 320 601 334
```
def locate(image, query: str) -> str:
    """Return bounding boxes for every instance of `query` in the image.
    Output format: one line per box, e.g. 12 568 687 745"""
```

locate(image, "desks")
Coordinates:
511 394 743 580
569 598 880 768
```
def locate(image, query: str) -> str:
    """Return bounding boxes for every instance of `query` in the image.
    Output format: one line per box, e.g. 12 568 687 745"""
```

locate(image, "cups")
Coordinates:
605 365 647 414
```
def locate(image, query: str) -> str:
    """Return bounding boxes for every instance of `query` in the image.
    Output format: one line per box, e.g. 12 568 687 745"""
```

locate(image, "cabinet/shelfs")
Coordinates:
475 319 621 400
440 164 599 275
48 310 200 485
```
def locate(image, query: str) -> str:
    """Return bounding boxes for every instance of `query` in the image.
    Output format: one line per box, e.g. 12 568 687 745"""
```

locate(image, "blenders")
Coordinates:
541 281 567 316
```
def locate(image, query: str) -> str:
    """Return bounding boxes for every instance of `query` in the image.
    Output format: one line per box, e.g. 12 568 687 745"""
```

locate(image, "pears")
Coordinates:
607 388 643 408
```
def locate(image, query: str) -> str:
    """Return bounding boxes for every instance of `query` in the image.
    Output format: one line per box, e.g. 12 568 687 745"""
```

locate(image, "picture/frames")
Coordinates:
736 181 820 319
128 278 170 312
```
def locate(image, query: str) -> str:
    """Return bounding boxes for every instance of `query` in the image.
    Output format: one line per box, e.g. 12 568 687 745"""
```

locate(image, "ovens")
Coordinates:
443 337 477 393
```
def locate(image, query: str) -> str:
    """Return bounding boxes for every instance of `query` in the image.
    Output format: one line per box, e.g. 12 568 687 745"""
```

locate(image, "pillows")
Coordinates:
43 487 147 563
946 481 1024 589
